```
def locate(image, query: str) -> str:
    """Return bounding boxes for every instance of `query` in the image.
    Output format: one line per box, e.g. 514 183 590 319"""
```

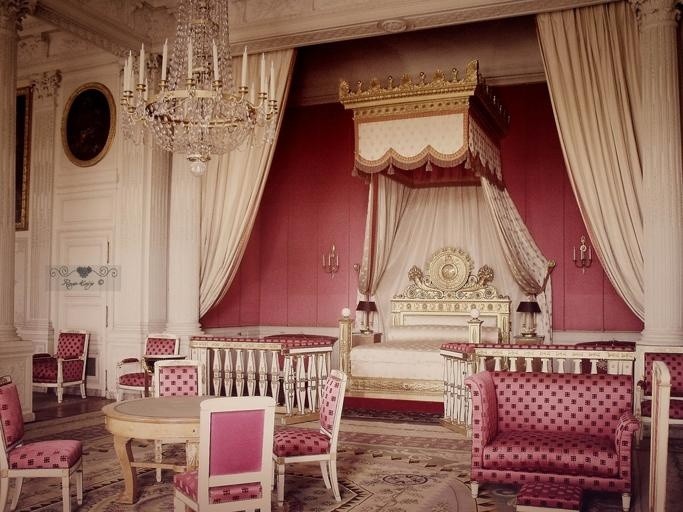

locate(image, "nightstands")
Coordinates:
513 335 545 344
351 332 382 348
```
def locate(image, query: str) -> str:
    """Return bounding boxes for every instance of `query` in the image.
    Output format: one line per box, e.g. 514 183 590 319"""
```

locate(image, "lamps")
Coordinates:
356 301 378 334
516 301 542 338
120 0 280 174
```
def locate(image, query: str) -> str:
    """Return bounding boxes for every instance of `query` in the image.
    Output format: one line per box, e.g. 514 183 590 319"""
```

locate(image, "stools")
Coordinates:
515 480 582 512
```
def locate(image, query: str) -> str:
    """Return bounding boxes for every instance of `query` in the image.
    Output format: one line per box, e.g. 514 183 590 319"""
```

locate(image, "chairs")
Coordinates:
0 375 85 512
154 360 205 482
114 334 181 401
635 344 683 441
174 396 274 512
29 329 90 403
272 368 348 507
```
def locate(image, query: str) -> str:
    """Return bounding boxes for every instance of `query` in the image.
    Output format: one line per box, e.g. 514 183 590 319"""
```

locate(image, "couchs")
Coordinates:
463 370 640 512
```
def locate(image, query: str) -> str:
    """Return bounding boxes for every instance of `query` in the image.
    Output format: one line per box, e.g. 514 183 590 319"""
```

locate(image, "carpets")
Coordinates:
0 420 679 512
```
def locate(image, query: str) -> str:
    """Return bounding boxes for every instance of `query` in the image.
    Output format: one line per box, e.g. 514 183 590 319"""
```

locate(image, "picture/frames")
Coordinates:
16 85 33 232
60 82 116 168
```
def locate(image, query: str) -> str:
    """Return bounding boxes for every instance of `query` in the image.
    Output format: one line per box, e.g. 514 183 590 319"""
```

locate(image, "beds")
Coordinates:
338 246 512 413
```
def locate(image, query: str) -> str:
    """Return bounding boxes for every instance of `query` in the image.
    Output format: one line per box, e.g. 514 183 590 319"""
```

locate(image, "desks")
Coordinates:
102 395 234 504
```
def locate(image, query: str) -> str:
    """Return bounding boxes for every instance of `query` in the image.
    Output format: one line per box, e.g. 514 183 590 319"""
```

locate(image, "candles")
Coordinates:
589 246 591 260
336 255 339 266
581 247 583 261
328 253 331 267
573 245 577 261
322 253 326 267
